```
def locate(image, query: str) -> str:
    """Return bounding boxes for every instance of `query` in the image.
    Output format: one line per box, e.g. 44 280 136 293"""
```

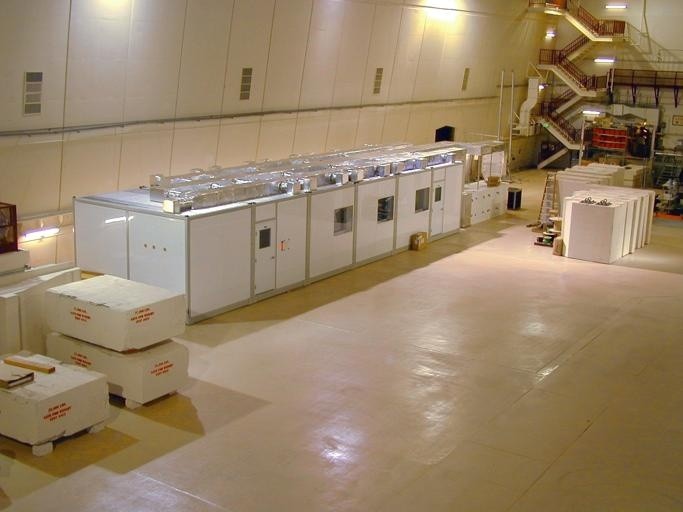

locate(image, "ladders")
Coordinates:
537 170 557 225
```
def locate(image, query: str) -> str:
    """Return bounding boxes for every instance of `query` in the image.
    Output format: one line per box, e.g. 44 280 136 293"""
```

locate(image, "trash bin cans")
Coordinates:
507 188 522 210
